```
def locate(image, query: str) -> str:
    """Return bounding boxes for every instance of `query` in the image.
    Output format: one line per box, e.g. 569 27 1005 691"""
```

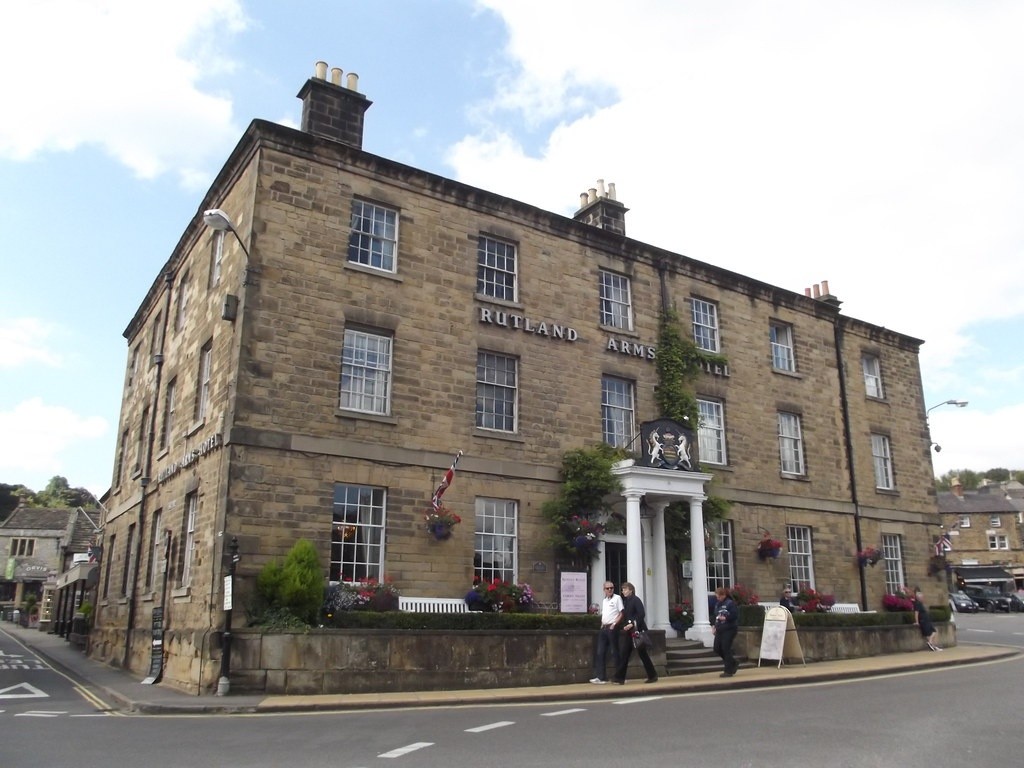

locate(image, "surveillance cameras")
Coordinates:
935 446 941 452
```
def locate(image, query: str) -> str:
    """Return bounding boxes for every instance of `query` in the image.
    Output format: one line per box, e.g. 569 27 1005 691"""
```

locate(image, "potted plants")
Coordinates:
25 595 39 628
79 601 93 635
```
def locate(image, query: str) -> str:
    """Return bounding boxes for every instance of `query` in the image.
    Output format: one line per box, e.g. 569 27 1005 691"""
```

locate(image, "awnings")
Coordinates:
955 565 1013 583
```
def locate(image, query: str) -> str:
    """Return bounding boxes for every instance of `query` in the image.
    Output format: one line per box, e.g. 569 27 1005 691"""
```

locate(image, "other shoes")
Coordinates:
733 661 739 673
611 676 624 685
926 641 935 650
720 673 732 677
589 677 607 684
646 676 659 683
934 647 942 651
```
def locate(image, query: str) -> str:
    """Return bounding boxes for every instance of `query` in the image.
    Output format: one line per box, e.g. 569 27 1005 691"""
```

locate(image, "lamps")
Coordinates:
203 209 249 260
640 494 657 519
681 414 690 425
338 519 356 542
926 400 968 417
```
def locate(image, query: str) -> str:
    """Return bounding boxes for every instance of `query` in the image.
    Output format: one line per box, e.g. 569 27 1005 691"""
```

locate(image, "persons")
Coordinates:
589 580 625 685
611 581 658 684
711 586 739 677
780 587 802 613
914 589 942 651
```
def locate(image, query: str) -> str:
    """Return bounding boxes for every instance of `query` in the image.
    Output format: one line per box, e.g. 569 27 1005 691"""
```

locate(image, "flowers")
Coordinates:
928 555 952 574
423 506 461 538
560 514 605 563
857 547 884 567
685 525 716 554
757 539 784 550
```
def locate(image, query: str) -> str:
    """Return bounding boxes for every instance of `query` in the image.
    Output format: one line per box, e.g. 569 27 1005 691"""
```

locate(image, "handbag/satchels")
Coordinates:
632 620 652 649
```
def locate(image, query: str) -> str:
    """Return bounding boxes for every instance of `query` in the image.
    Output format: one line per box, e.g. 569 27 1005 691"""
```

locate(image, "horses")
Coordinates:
645 430 664 464
675 433 692 468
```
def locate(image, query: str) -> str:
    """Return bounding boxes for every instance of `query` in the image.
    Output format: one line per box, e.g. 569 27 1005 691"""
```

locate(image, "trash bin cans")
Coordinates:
13 610 19 622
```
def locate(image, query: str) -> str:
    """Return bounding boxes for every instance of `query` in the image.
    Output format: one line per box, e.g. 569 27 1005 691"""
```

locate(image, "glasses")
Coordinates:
605 587 613 590
785 591 791 593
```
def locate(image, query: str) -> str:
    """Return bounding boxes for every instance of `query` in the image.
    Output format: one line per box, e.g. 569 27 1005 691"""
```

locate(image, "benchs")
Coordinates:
396 595 483 613
817 604 878 613
757 601 781 613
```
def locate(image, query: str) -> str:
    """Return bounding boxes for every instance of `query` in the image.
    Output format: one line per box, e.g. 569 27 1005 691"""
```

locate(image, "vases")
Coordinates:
863 558 877 565
757 548 783 560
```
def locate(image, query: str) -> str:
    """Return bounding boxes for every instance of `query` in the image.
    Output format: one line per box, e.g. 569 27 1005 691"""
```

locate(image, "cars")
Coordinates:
948 585 1024 613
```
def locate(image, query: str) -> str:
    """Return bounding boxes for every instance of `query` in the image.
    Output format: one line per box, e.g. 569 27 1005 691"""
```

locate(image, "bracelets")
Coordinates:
613 622 616 626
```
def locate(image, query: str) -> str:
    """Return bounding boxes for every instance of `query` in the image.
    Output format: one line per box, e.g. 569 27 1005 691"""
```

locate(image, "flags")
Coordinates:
935 532 951 556
87 541 97 564
431 449 463 513
